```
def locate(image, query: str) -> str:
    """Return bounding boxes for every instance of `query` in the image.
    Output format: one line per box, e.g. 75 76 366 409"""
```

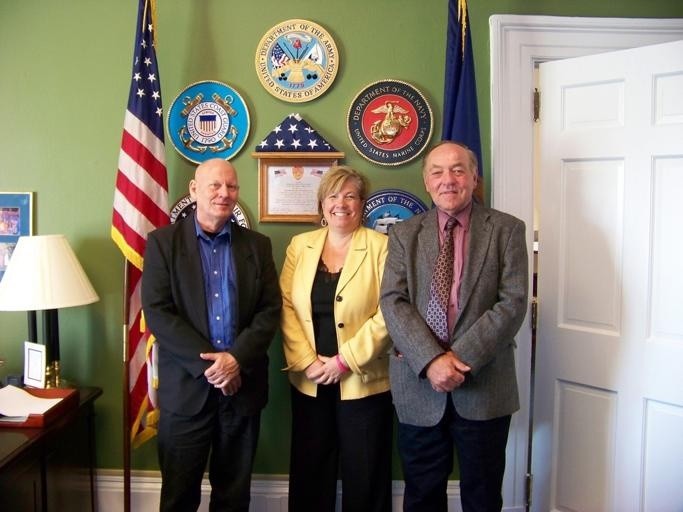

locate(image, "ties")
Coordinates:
425 218 459 351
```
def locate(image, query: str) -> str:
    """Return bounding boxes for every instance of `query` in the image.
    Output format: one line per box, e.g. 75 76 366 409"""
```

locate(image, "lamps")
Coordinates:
0 235 102 391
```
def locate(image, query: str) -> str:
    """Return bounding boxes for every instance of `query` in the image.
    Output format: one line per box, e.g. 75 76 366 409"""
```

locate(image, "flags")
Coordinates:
110 0 172 449
432 0 485 211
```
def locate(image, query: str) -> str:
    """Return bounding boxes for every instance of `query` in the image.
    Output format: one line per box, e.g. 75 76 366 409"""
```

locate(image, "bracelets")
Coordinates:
336 354 350 373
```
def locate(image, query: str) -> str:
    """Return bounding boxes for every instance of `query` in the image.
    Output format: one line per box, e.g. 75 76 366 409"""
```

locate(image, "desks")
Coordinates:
1 386 102 512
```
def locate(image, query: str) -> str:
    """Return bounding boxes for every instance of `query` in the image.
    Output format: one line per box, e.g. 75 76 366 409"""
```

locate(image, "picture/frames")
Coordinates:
0 191 33 283
251 152 343 223
24 340 46 389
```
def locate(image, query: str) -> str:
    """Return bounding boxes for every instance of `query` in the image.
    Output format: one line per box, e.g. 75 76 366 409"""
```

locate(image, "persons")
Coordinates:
379 140 529 512
278 166 394 512
142 158 283 512
